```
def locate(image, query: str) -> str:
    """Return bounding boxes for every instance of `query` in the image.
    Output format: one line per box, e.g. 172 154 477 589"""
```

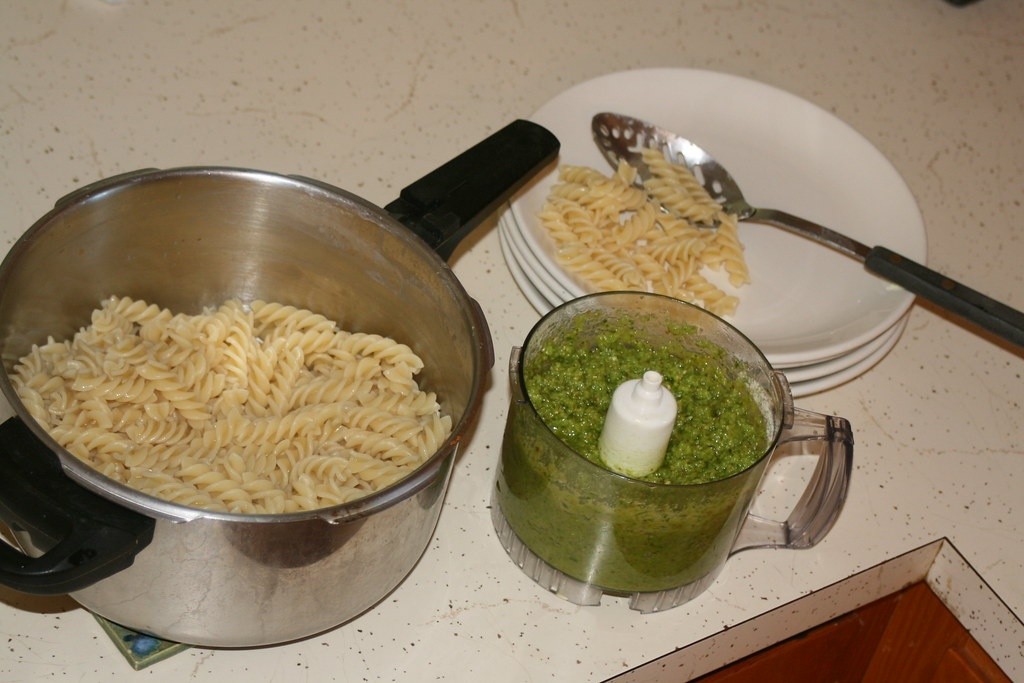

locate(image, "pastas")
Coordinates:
535 146 749 321
4 293 453 512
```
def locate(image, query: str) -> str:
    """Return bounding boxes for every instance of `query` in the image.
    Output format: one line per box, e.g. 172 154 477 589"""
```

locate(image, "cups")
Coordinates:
489 290 854 615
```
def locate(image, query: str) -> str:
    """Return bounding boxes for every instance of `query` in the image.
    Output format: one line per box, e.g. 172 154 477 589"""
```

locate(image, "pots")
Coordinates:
0 117 561 651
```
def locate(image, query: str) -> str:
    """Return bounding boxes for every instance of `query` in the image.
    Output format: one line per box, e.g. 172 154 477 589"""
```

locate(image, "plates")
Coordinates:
498 67 927 397
